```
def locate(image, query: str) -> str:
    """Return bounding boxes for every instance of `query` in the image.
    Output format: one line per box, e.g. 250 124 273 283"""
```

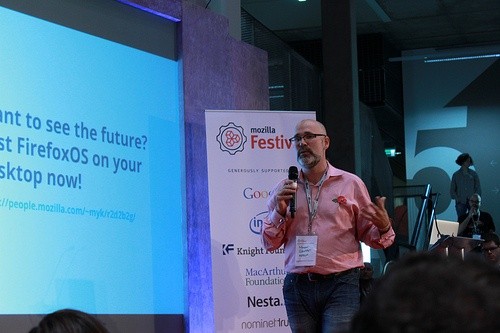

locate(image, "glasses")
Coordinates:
288 133 327 143
482 246 499 252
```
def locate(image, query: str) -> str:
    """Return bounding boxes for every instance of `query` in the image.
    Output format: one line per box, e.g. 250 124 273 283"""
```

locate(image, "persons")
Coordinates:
28 234 500 332
260 119 395 333
450 153 481 222
457 193 495 242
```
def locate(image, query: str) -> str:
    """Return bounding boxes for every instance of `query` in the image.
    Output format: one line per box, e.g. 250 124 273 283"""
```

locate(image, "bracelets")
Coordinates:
378 222 390 234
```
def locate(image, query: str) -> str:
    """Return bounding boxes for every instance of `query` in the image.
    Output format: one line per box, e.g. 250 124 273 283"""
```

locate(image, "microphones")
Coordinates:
288 166 298 218
